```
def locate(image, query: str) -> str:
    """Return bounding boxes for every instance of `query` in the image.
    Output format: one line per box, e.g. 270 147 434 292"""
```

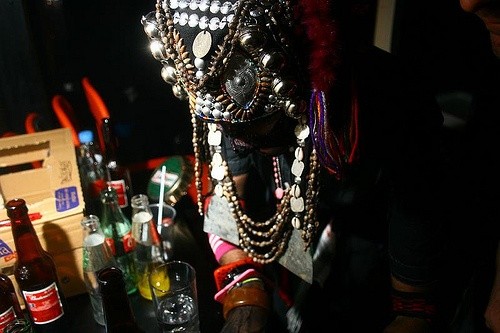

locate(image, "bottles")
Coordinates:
5 199 70 333
76 130 169 333
0 273 25 333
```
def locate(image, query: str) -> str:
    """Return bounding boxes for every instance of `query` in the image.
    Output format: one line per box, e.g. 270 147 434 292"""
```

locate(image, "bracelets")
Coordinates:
221 288 267 319
214 258 268 302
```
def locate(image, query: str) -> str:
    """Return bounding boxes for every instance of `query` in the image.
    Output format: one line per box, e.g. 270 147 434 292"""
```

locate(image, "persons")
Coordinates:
142 0 500 333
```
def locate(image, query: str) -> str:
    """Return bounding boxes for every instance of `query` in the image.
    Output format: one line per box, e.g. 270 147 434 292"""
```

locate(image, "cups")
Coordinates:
150 204 177 261
148 261 200 333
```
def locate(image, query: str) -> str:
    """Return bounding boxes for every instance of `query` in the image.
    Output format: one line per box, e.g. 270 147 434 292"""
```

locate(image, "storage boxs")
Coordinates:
0 128 97 310
